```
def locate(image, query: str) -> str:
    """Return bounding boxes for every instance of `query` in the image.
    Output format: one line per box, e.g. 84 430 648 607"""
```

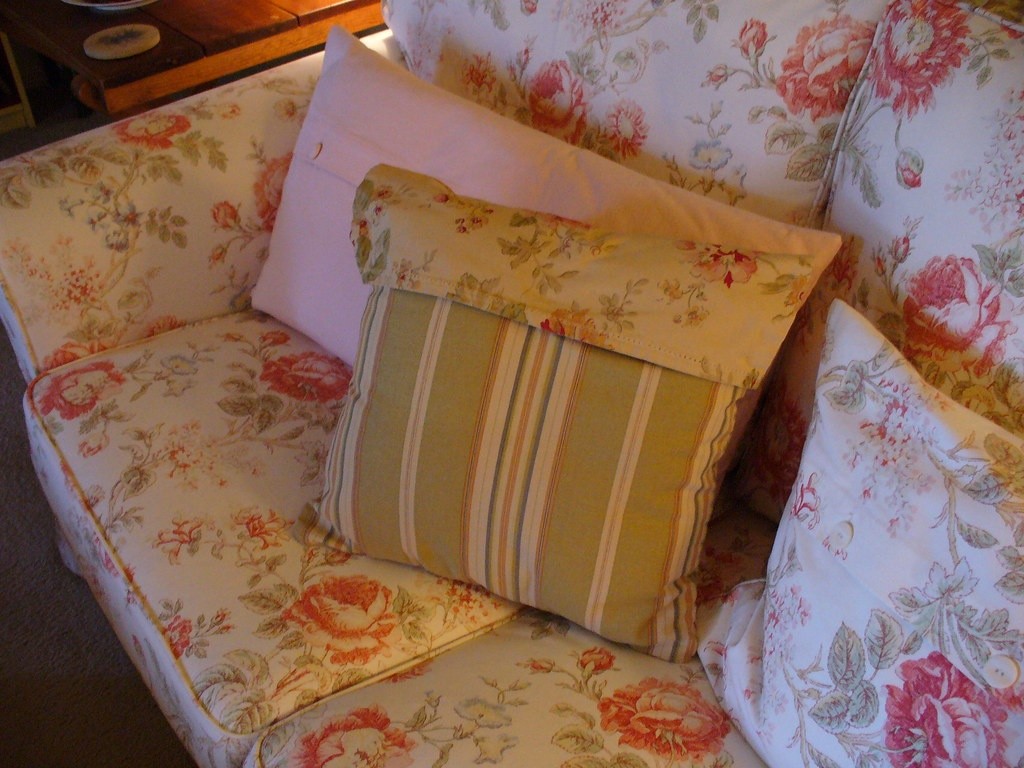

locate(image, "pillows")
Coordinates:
248 23 842 373
287 160 816 663
696 295 1023 767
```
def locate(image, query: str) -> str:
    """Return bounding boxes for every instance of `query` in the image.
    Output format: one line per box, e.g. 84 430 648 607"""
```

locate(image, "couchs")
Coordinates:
0 0 1024 768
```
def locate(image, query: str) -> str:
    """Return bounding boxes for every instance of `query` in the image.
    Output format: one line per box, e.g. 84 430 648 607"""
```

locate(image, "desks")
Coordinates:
0 0 387 122
